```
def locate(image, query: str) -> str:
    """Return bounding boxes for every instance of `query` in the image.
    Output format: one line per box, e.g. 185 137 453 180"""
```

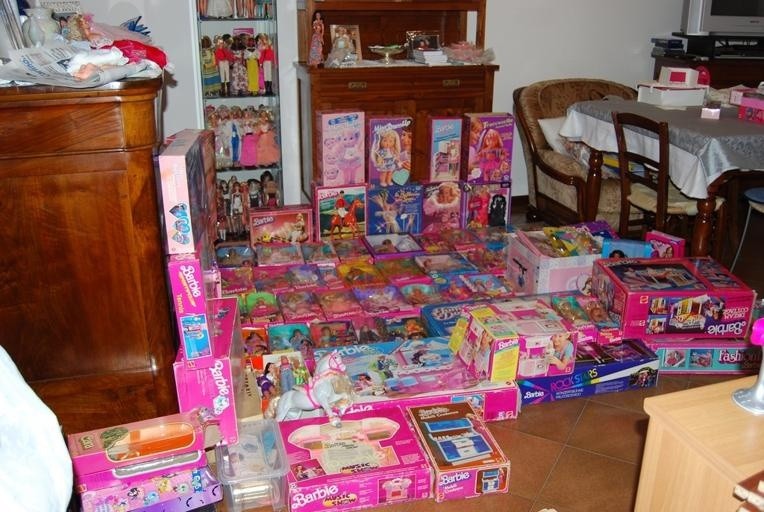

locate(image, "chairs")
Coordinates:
512 78 637 227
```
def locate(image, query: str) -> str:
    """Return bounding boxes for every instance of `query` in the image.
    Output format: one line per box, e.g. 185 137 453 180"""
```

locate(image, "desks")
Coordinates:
0 77 181 444
651 53 764 90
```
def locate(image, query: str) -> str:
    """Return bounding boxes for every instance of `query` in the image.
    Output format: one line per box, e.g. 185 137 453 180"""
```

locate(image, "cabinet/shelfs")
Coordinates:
189 0 302 249
292 0 500 212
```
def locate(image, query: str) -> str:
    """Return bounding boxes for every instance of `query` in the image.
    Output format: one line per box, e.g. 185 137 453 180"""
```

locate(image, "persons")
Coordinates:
332 191 349 219
309 10 324 68
325 25 357 68
551 335 573 370
199 29 278 241
417 38 432 48
60 15 67 27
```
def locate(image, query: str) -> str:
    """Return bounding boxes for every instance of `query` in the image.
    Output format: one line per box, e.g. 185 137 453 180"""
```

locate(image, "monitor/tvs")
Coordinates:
680 0 764 36
